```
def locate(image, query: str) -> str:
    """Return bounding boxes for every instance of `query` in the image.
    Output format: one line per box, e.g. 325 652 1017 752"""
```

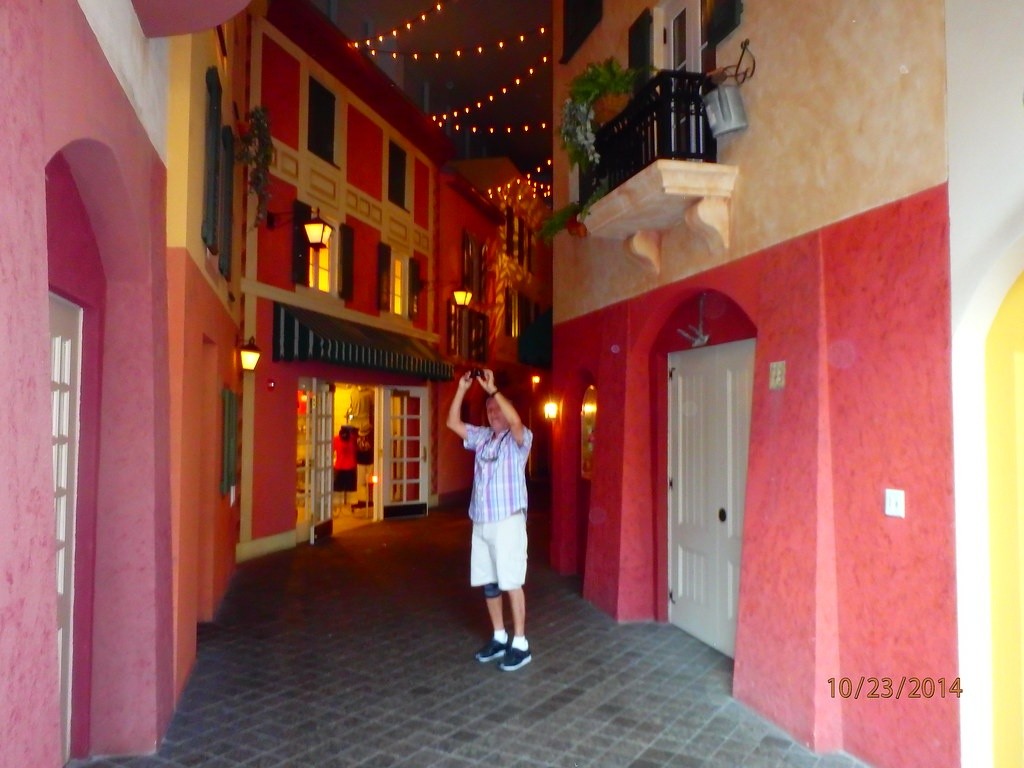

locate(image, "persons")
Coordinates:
445 369 533 671
331 424 358 492
355 423 374 503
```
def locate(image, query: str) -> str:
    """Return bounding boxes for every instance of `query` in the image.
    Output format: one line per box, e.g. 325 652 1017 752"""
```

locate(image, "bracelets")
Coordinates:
491 390 500 397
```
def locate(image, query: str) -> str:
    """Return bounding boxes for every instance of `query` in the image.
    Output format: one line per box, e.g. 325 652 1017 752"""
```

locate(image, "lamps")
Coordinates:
305 208 333 248
240 337 262 370
454 280 473 309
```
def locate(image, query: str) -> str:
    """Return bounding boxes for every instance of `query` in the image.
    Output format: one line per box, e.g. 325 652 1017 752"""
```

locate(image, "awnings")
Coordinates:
272 302 453 383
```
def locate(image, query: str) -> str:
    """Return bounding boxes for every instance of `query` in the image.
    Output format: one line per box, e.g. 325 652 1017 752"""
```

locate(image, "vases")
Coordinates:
238 119 253 135
592 93 631 125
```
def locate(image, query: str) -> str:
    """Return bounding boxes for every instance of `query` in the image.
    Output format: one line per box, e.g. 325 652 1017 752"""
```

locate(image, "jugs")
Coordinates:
699 68 747 139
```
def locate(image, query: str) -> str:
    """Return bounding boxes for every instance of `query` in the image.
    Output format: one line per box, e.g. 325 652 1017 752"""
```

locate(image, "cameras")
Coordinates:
469 369 483 379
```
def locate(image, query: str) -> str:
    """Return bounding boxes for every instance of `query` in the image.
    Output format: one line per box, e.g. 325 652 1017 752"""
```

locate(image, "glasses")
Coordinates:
481 438 502 462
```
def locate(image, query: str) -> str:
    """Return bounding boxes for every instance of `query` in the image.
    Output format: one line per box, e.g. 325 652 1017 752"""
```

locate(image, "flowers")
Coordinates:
557 58 654 172
244 104 277 192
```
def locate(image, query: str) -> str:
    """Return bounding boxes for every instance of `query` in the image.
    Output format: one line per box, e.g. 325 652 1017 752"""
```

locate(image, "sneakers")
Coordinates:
497 641 532 672
475 633 510 663
